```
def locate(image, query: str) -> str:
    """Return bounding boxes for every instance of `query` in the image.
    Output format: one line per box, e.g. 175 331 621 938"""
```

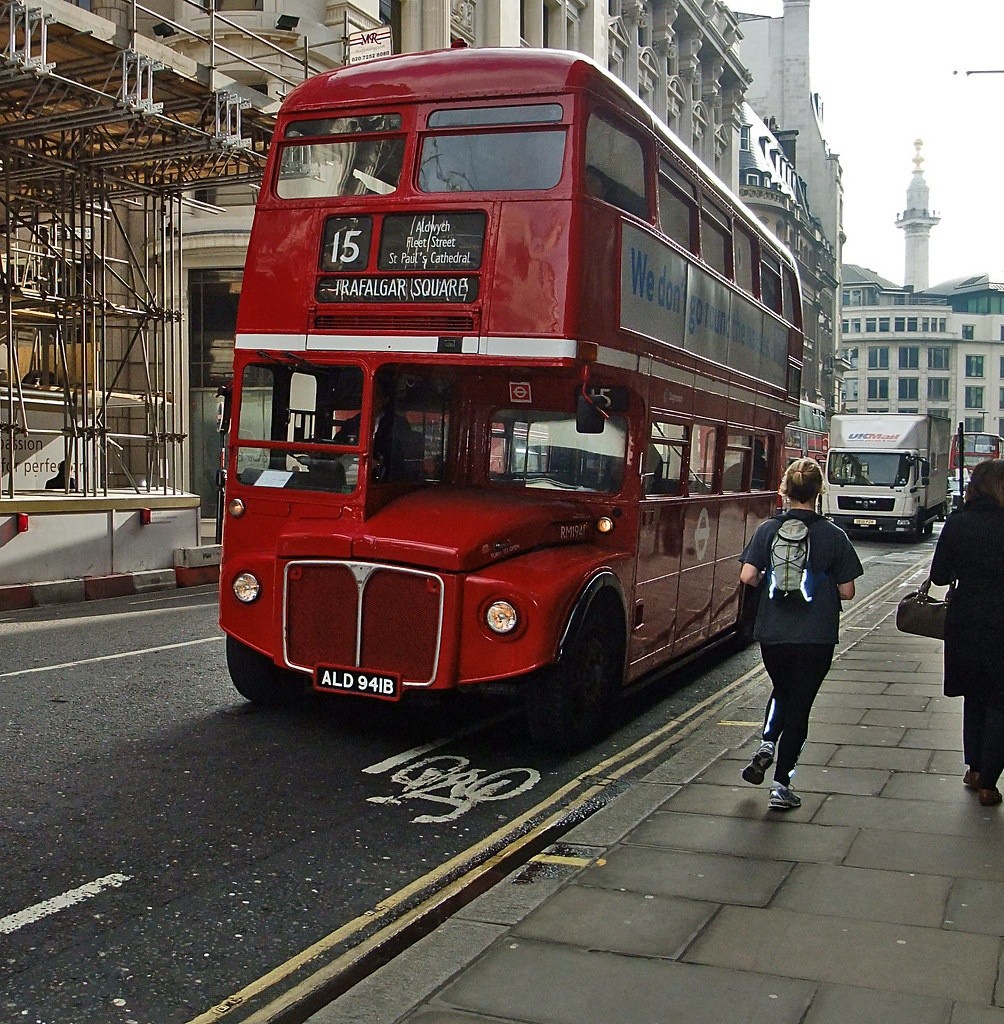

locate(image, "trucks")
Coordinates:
820 413 952 545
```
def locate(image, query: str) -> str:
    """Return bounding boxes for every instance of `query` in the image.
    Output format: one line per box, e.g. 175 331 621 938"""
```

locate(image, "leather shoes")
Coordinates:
978 788 1002 806
963 770 981 787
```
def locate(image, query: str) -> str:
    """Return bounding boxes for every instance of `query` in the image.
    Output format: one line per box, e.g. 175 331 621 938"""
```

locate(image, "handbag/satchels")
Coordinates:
895 576 956 640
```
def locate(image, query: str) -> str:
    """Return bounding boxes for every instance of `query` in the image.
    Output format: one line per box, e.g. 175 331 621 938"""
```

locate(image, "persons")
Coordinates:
929 458 1004 806
44 460 76 489
330 376 415 482
750 438 767 489
583 160 614 202
737 456 864 810
601 455 624 493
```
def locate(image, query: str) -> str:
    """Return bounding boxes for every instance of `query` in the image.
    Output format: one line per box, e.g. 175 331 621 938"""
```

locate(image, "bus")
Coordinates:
218 48 806 733
782 397 828 469
949 431 1004 478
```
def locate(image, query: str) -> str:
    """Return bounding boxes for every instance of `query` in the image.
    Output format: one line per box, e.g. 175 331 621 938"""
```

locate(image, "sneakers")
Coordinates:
741 741 776 785
767 785 803 808
